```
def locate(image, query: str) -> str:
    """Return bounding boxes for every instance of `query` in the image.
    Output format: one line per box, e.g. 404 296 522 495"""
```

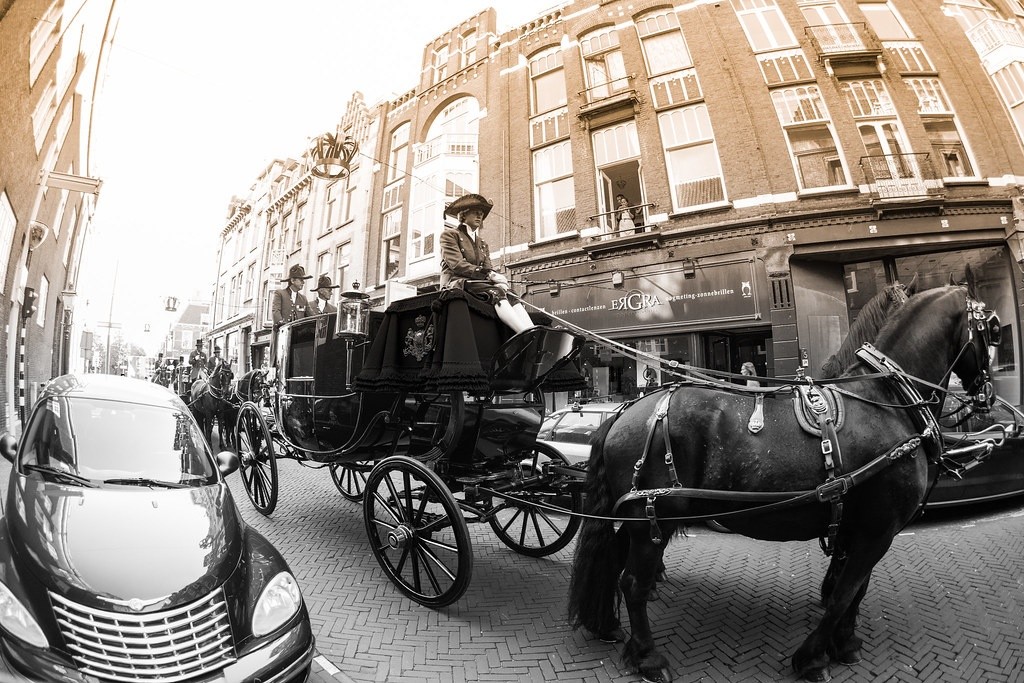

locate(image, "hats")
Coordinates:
179 357 184 362
159 352 163 357
310 276 340 292
196 340 203 346
442 194 494 220
213 347 221 353
280 265 311 283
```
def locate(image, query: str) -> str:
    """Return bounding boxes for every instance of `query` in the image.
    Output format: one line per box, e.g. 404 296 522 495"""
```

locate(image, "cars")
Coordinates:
0 370 321 683
915 386 1023 515
514 402 633 514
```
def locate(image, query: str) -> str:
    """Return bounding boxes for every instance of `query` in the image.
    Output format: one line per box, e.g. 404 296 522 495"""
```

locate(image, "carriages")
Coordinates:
234 270 1004 683
170 358 239 455
148 358 181 392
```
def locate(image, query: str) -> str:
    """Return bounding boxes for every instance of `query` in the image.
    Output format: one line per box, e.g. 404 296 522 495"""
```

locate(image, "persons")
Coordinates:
189 339 207 383
308 277 337 316
155 353 164 370
207 346 228 376
121 373 125 377
617 198 635 237
175 356 186 375
740 362 760 388
269 263 316 367
616 193 642 221
439 193 536 334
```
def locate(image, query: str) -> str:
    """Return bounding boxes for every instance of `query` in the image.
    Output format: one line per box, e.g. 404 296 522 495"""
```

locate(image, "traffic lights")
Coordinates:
22 287 38 319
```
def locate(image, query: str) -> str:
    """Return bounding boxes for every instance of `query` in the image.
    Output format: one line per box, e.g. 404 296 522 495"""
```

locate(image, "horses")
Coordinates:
152 366 169 388
191 359 234 452
567 262 998 683
224 369 270 452
